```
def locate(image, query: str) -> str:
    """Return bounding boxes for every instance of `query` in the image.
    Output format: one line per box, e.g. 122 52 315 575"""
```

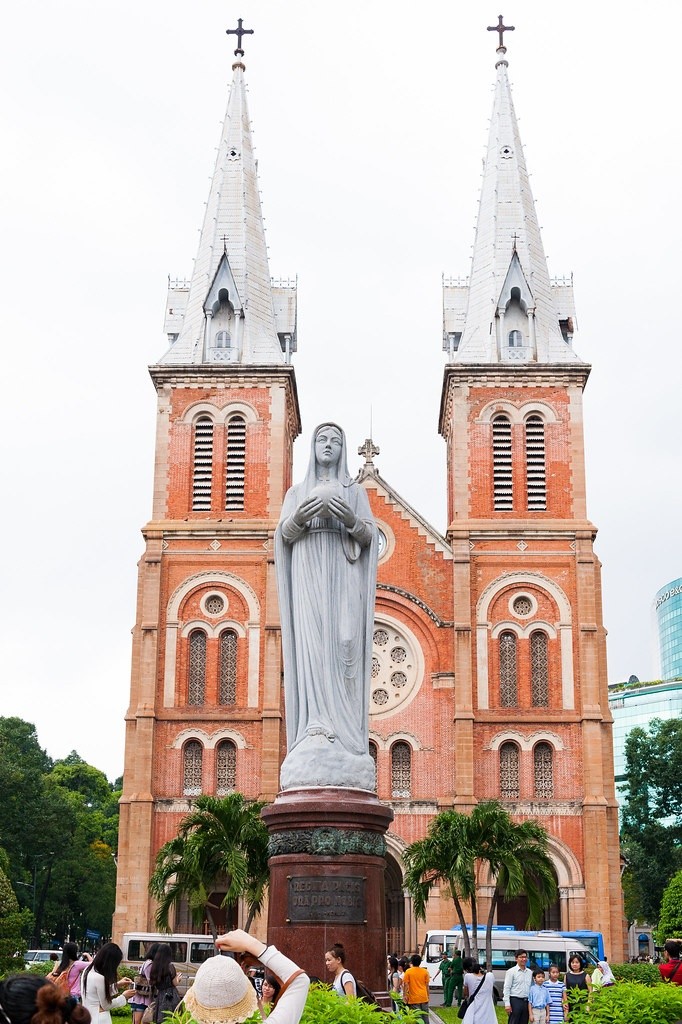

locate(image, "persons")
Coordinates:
542 964 569 1024
562 955 592 1012
273 421 380 755
503 949 534 1024
0 929 322 1024
528 968 553 1024
632 938 682 985
324 943 357 1000
591 961 616 990
461 957 499 1024
387 950 463 1024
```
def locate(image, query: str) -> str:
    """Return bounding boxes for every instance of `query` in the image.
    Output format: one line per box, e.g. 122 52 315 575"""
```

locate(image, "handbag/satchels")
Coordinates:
357 981 375 1004
134 960 152 996
142 1002 155 1022
458 1000 468 1019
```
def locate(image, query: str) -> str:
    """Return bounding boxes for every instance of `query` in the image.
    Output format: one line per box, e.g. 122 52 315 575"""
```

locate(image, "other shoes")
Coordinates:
440 1003 445 1006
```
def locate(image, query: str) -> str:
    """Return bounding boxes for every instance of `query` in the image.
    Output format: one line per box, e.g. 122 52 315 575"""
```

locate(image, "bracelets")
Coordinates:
256 942 268 959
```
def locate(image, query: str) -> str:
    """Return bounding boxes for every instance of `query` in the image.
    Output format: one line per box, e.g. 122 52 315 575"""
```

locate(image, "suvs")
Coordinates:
12 950 64 971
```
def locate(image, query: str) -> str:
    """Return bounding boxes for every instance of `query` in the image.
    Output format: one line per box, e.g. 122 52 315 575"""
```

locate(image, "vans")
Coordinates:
121 932 224 998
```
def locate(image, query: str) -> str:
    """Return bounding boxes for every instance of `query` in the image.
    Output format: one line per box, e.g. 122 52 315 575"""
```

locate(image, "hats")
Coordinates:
441 952 447 955
184 954 259 1024
455 949 461 955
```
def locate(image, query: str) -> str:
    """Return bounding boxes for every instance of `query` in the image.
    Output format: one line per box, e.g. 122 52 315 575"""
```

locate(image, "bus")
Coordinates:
419 930 604 1004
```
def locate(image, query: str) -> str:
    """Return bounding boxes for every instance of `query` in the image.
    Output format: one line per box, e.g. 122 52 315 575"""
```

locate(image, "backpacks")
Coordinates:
45 964 81 996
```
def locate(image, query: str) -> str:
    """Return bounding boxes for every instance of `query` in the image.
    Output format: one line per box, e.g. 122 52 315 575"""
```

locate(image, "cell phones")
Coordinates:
130 982 135 990
178 973 183 977
79 956 86 961
219 949 234 959
